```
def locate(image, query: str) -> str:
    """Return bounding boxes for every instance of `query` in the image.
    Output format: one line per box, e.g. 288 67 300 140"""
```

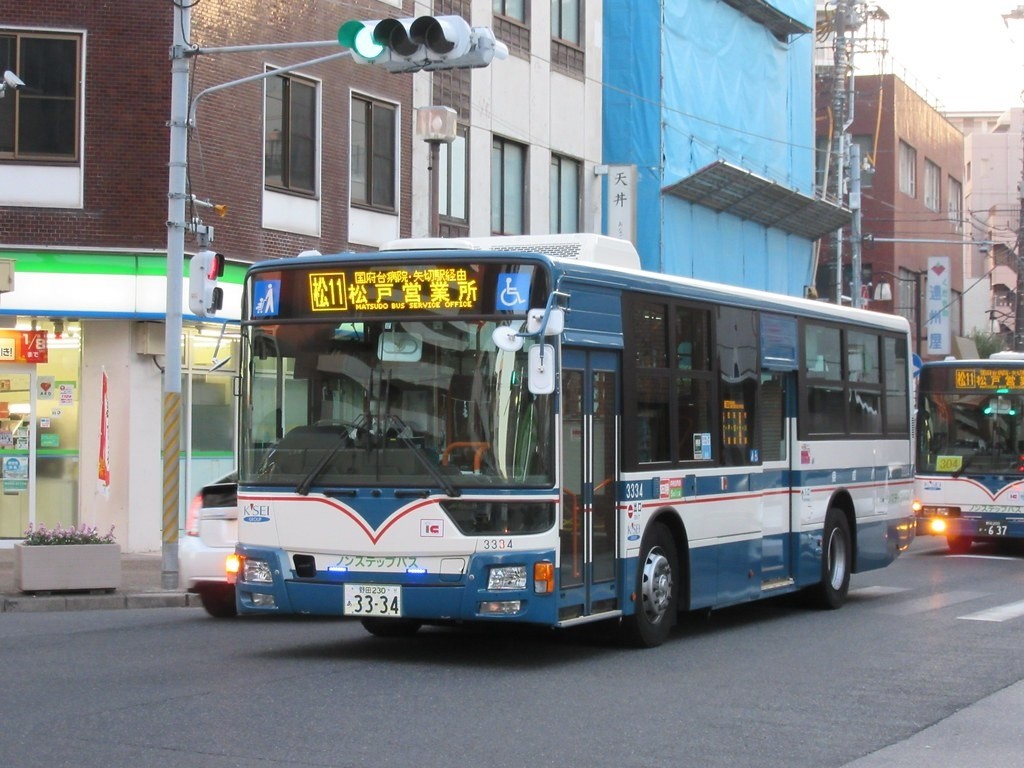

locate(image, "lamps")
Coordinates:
872 273 893 302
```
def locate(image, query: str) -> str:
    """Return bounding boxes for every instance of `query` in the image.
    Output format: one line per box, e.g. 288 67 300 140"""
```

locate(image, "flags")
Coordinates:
98 366 111 488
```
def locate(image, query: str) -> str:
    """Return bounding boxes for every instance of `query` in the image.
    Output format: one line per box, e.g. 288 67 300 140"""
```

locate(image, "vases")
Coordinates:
15 544 120 590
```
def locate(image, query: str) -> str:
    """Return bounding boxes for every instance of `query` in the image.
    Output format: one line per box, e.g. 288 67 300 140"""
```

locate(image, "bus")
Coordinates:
910 349 1024 557
235 233 922 646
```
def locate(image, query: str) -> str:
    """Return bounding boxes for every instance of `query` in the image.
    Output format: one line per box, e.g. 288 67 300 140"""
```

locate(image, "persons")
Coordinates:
348 383 413 441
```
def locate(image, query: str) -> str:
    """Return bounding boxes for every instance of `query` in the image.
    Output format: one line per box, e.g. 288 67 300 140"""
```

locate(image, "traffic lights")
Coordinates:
333 12 475 71
189 245 229 318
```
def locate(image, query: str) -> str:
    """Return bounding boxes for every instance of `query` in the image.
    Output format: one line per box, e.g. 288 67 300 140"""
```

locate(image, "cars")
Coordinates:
182 471 238 619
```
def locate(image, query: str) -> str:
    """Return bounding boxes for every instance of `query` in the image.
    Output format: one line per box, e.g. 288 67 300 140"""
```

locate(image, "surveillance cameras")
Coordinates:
4 70 26 90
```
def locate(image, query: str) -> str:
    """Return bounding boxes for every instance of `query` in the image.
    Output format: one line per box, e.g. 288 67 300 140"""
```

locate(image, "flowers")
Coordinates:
21 519 115 545
